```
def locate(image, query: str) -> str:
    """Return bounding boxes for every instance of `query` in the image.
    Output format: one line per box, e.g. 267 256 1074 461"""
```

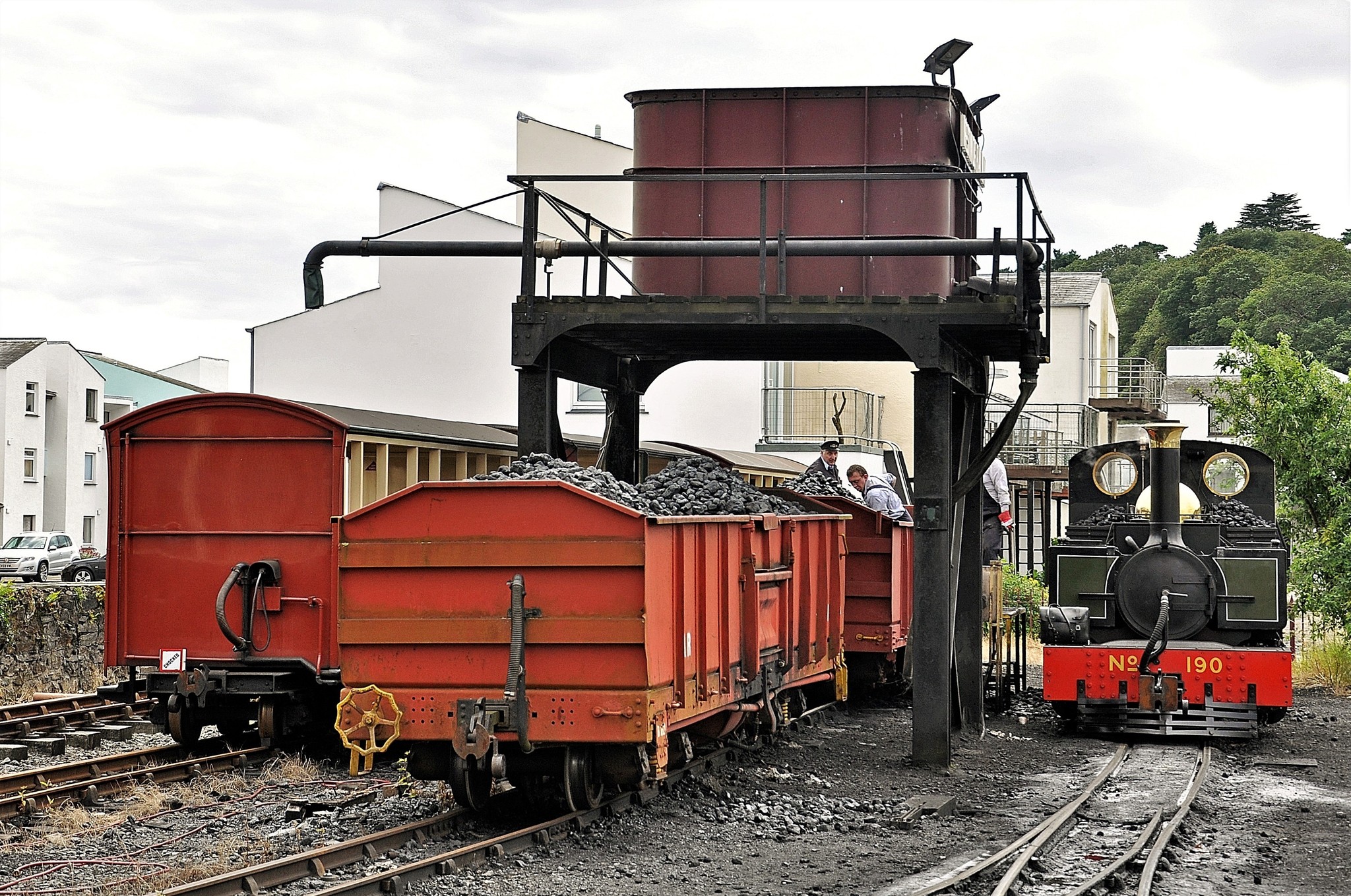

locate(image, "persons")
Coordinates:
847 464 914 522
981 458 1016 566
805 440 842 486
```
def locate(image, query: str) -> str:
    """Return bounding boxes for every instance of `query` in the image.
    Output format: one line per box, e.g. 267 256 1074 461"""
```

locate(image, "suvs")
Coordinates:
0 531 81 583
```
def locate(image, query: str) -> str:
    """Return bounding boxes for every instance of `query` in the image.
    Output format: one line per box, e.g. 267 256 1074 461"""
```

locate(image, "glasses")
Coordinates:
848 474 864 486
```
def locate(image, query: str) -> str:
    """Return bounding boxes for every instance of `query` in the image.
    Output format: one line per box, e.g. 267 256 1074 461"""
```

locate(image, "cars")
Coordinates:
61 554 106 582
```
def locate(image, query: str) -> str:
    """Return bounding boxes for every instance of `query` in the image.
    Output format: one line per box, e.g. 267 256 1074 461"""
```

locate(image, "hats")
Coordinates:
819 440 840 451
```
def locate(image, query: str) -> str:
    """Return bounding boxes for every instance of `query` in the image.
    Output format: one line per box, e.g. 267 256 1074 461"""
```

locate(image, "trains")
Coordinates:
1043 423 1296 737
333 486 915 815
99 394 816 749
